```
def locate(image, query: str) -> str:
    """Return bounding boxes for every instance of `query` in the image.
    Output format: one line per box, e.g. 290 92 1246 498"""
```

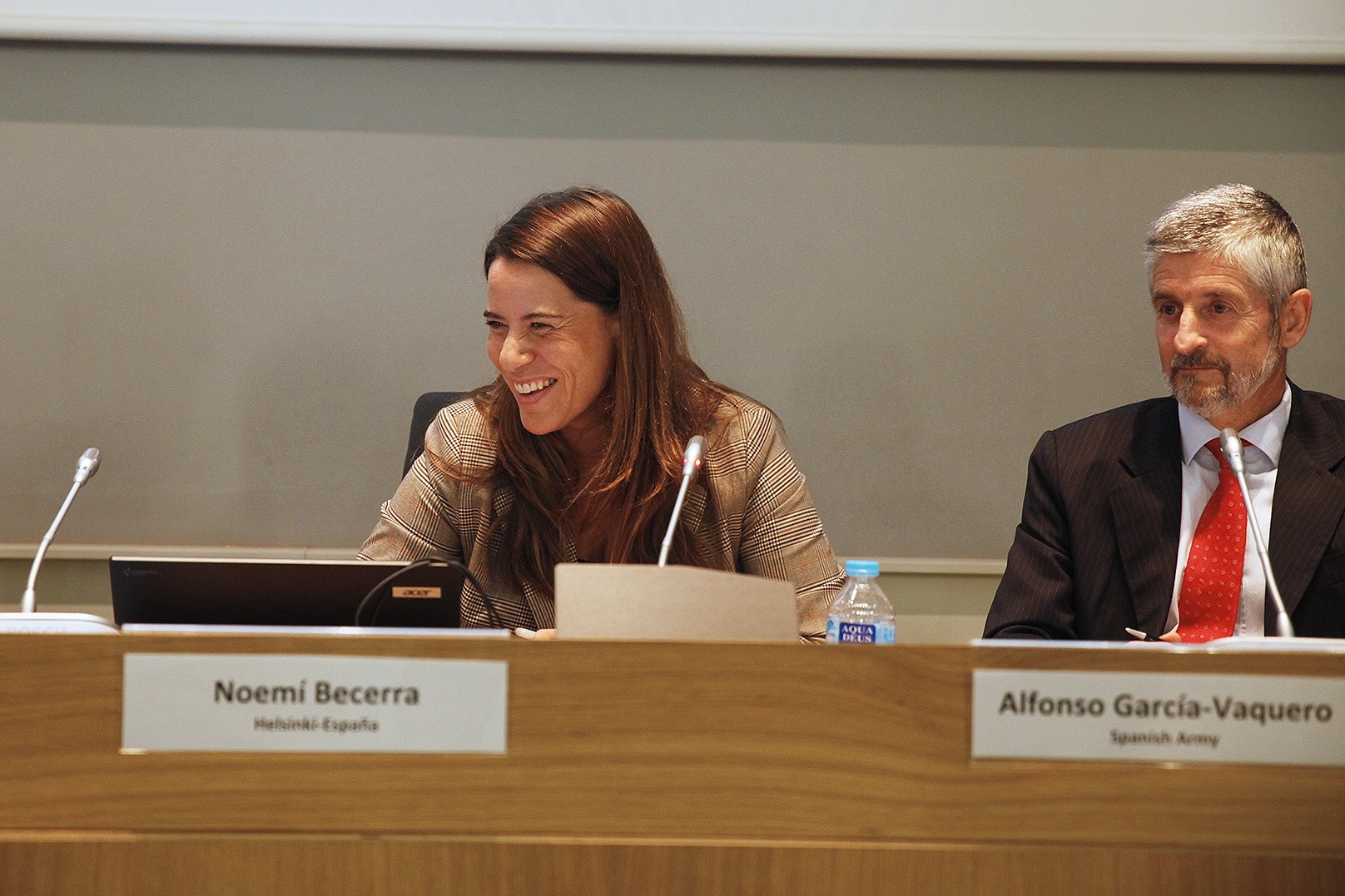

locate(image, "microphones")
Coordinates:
0 448 119 634
1207 427 1345 652
659 435 706 565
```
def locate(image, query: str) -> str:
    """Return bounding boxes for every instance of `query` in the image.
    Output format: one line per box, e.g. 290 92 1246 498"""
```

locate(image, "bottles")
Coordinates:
826 560 897 644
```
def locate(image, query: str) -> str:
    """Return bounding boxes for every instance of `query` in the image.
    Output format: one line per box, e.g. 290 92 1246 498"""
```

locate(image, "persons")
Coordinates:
979 180 1345 642
352 175 852 644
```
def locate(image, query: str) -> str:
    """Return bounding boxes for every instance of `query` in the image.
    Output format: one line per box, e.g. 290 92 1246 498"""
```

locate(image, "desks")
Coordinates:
0 633 1345 896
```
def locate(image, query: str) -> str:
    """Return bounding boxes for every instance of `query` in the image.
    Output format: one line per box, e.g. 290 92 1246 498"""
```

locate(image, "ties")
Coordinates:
1176 436 1252 643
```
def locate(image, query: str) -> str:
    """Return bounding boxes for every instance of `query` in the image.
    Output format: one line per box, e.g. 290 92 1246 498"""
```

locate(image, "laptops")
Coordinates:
107 557 464 631
